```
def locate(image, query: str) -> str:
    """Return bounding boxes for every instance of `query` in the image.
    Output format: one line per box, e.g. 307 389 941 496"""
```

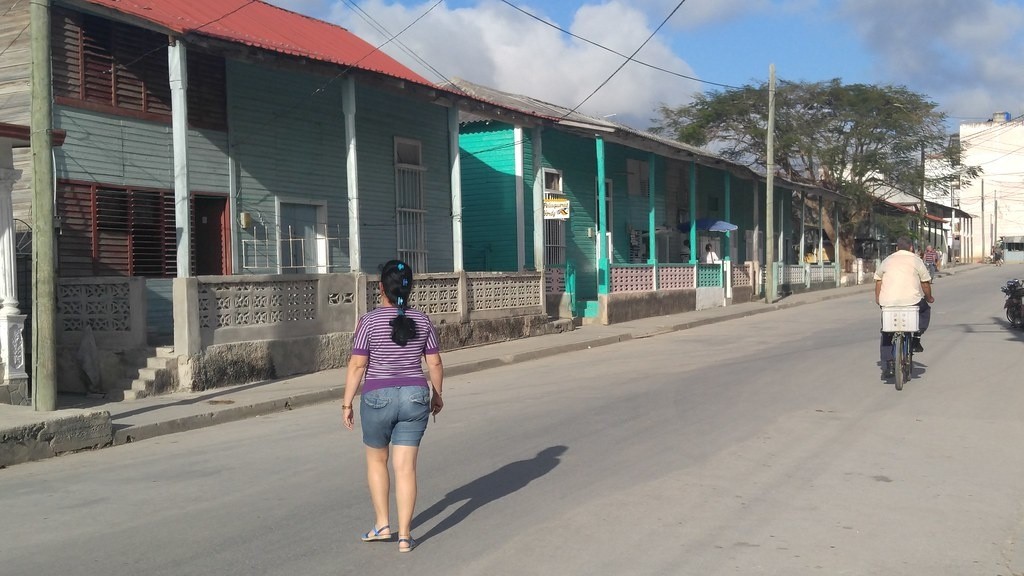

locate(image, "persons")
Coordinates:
915 242 954 283
339 256 445 554
699 244 721 263
681 238 690 262
873 233 938 376
990 243 1005 267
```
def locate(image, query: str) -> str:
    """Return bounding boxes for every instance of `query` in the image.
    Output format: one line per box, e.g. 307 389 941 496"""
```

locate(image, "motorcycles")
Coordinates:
1001 279 1024 331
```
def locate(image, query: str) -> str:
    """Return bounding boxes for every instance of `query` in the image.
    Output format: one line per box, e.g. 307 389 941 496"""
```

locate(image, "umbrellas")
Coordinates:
675 217 738 242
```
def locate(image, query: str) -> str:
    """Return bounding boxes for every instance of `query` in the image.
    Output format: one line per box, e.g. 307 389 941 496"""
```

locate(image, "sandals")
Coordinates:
397 535 417 552
361 525 392 541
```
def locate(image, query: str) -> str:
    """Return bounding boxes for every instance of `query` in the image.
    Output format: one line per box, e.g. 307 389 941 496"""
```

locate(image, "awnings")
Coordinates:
925 200 979 219
1003 236 1024 244
873 195 950 223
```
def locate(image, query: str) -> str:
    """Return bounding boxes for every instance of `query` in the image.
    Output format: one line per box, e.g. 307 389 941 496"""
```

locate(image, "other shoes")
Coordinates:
883 360 895 379
911 339 923 352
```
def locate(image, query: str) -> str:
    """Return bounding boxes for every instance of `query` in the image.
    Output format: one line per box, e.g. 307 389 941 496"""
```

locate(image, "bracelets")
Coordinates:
341 404 352 410
432 391 443 396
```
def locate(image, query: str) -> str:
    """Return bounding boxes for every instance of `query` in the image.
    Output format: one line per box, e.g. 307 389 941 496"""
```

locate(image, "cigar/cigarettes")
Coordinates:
433 409 436 423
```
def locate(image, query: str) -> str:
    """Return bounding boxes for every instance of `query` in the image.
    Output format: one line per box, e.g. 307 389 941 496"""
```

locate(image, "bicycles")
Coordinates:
878 295 933 391
924 263 935 283
